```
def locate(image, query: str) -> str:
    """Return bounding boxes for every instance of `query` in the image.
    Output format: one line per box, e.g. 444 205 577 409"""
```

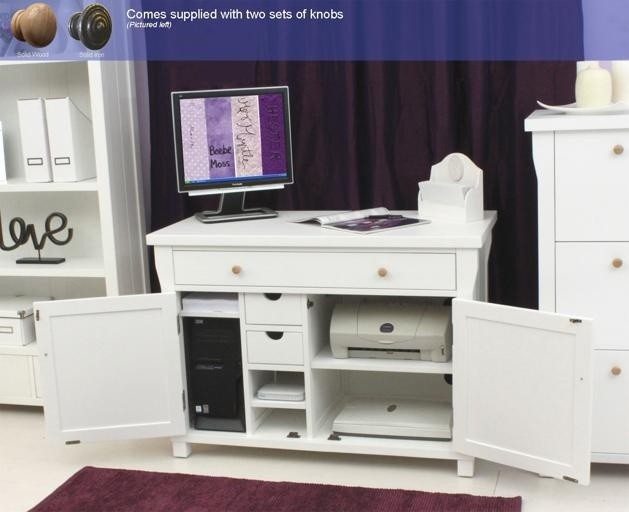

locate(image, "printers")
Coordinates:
329 295 452 363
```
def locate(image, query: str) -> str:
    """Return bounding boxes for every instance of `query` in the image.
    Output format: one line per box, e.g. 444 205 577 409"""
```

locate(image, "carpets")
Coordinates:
32 464 523 511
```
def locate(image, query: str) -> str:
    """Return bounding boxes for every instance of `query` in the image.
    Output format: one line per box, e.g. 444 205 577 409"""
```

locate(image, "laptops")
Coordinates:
333 396 452 440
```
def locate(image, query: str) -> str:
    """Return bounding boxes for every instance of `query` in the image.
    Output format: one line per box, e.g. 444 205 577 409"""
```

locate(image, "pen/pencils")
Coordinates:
369 215 402 219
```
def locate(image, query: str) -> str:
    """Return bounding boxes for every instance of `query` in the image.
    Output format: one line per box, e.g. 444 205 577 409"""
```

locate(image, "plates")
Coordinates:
535 98 628 115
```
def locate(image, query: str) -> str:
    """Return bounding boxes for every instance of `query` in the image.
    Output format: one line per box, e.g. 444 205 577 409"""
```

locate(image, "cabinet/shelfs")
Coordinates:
524 102 629 465
35 207 599 490
0 58 152 413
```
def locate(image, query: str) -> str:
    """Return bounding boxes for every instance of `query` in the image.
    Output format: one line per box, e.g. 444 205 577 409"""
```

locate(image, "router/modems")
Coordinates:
257 371 305 402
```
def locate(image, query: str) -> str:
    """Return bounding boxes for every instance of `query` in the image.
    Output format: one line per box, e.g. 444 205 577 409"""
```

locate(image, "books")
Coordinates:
288 206 433 235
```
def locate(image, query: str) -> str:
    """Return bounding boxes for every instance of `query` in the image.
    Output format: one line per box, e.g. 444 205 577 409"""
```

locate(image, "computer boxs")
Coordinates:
183 317 246 433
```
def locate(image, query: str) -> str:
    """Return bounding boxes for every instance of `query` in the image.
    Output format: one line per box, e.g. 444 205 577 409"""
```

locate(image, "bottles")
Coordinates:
573 59 613 105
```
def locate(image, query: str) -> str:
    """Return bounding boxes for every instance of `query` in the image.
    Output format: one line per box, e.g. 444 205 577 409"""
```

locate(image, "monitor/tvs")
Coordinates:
171 85 295 223
